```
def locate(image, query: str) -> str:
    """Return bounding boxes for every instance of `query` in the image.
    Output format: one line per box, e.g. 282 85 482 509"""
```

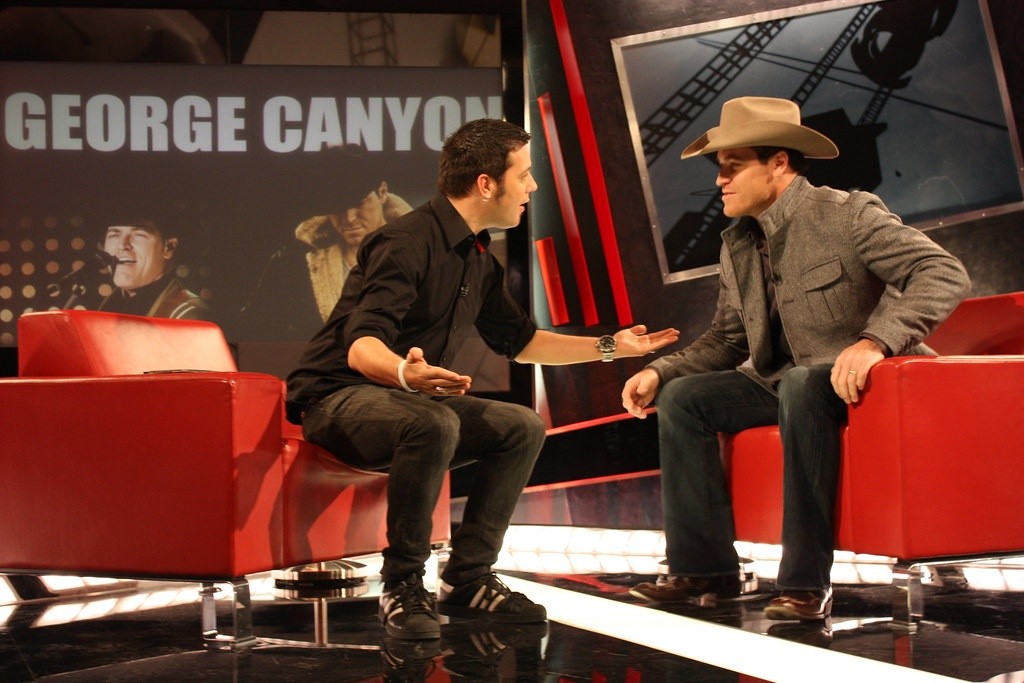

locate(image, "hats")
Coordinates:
300 143 391 213
677 95 842 159
92 194 209 264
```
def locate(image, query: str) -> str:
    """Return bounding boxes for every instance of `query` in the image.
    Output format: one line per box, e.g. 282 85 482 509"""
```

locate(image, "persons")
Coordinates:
621 98 972 619
86 194 215 325
285 118 681 637
258 142 417 369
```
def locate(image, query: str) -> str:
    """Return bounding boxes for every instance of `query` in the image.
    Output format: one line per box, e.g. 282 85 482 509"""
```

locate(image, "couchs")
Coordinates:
0 309 450 645
656 295 1024 631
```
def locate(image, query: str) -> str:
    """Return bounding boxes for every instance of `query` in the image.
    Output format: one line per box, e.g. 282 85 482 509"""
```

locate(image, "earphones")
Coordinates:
166 243 174 252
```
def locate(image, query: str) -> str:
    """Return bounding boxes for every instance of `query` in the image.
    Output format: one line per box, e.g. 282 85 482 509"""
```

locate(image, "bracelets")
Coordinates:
397 358 419 392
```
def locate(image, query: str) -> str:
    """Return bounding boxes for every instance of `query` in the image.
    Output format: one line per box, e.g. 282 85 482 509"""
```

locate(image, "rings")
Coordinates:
849 370 857 374
436 386 447 394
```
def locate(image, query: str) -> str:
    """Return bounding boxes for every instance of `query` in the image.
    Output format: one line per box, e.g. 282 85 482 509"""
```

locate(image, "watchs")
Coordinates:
594 334 617 362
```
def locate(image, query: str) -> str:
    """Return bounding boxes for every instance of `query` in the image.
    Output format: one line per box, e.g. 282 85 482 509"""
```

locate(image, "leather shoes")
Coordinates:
630 567 744 604
764 581 835 622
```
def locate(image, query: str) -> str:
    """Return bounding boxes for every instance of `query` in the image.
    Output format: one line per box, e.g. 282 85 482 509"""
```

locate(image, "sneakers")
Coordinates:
377 578 441 640
440 620 549 664
434 571 548 624
377 637 442 683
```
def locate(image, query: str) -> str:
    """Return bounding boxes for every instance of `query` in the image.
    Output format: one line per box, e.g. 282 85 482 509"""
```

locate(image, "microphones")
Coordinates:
47 251 113 296
460 283 469 297
771 272 779 283
